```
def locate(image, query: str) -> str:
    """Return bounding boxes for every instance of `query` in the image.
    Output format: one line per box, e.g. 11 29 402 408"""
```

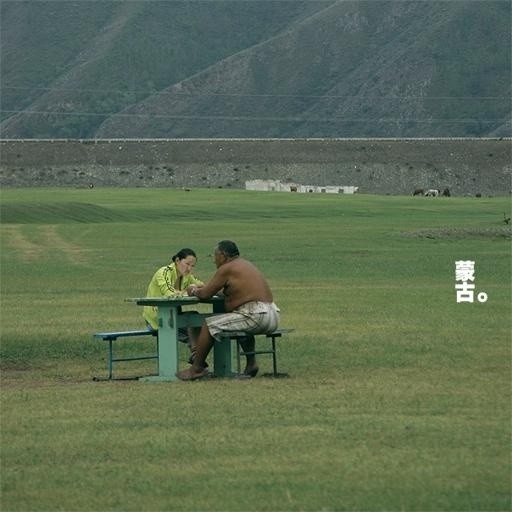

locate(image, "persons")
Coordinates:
142 249 209 368
174 244 280 380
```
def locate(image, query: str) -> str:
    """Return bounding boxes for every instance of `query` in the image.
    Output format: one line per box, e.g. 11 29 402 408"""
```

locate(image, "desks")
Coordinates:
125 297 251 383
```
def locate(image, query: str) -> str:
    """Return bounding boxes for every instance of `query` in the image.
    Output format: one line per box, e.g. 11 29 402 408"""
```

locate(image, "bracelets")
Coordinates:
190 288 196 295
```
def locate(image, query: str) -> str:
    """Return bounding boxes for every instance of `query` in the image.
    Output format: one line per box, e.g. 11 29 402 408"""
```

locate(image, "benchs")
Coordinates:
222 329 294 380
93 327 158 381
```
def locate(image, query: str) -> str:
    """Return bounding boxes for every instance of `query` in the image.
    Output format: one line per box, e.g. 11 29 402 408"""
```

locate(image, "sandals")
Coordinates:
188 350 208 368
